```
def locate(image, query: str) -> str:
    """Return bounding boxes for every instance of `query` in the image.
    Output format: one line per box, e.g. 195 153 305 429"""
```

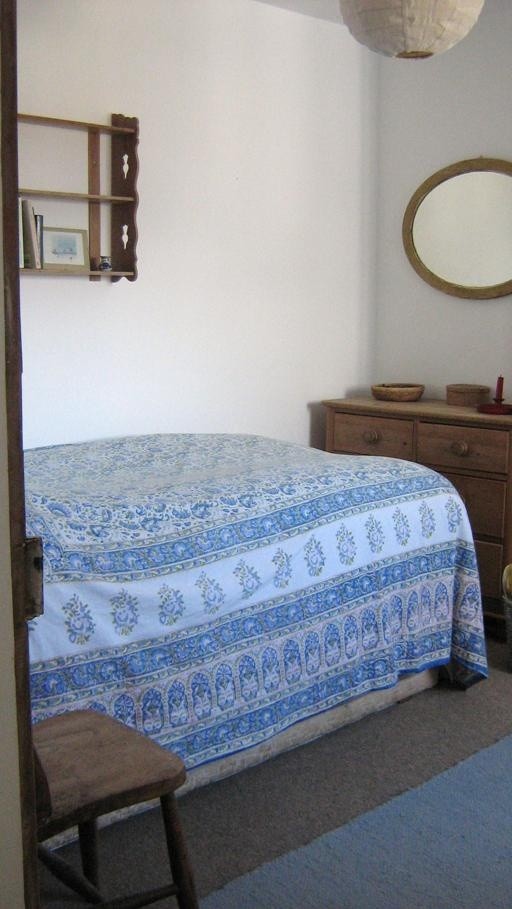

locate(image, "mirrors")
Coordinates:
399 153 512 302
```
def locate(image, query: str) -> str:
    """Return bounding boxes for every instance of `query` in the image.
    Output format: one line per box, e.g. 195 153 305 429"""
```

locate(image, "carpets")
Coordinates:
26 664 511 895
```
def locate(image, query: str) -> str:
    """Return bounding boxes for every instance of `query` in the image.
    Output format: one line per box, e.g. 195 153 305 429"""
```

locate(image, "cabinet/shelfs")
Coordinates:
317 395 512 639
16 115 141 286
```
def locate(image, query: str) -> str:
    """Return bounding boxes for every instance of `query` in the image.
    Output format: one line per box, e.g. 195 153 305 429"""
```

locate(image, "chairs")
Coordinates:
22 540 195 908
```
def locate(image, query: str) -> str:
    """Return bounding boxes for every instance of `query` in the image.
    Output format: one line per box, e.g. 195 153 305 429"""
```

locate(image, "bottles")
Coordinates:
99 255 112 272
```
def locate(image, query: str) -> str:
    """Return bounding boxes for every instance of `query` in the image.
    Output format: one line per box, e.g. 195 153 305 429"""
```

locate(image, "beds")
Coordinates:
22 429 490 862
